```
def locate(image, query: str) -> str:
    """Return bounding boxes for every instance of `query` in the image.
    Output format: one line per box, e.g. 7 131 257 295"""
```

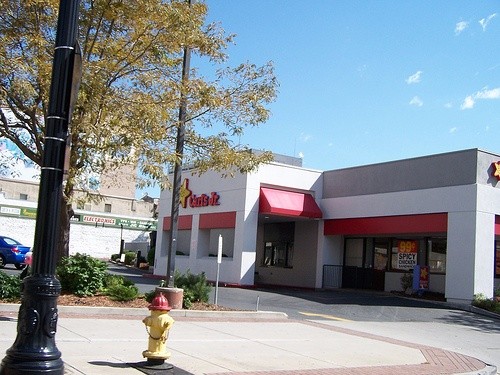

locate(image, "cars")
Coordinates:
0 236 31 269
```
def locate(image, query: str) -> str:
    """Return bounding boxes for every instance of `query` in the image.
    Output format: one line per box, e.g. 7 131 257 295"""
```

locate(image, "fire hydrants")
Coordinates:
142 293 175 365
